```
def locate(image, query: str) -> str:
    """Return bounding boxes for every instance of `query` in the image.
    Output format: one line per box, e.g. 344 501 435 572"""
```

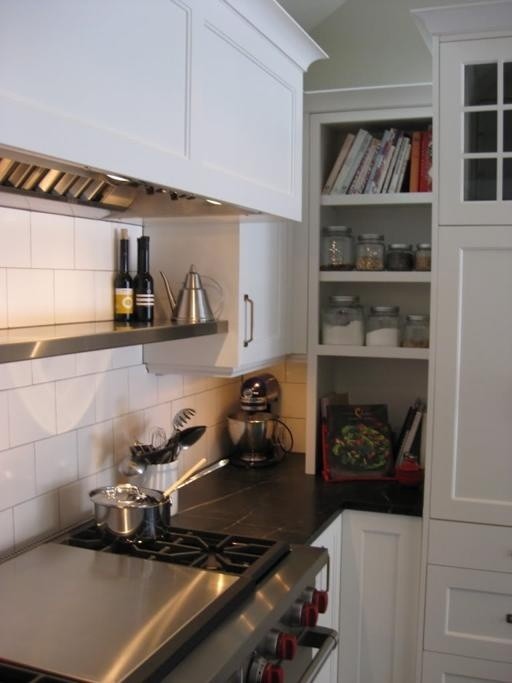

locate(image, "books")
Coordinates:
319 124 434 192
319 385 424 484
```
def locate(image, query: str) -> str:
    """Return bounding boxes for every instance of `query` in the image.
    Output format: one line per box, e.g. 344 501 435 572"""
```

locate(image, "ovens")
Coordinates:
251 620 340 683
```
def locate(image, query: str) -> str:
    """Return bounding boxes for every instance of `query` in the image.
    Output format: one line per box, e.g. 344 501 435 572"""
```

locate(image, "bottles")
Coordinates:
133 236 155 323
113 227 134 322
403 312 430 348
353 232 387 269
396 451 419 485
365 303 402 346
388 243 416 271
320 294 365 346
320 225 356 269
415 243 431 271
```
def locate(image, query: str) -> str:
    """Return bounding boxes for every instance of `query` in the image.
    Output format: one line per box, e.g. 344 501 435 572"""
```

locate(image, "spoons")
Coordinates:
179 425 206 449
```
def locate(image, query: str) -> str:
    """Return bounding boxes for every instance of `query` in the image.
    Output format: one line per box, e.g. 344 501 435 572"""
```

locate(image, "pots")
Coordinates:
87 455 230 540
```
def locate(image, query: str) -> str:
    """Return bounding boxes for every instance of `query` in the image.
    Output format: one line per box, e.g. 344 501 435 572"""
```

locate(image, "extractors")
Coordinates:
0 156 137 211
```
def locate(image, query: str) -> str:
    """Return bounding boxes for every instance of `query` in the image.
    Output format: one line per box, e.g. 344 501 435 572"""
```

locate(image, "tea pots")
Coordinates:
159 262 226 321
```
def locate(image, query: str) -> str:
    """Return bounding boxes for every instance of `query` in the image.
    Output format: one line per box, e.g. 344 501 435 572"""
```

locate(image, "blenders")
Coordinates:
226 374 293 469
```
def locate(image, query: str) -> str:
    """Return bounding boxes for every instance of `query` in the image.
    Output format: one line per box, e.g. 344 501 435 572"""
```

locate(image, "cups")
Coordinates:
128 458 178 518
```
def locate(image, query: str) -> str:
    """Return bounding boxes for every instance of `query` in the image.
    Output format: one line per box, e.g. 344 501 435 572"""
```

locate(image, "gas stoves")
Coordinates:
1 518 334 683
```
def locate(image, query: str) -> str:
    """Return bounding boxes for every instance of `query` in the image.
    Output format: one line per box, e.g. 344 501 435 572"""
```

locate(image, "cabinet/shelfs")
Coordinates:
1 0 333 225
134 210 305 377
301 514 343 682
301 79 438 476
408 1 512 682
